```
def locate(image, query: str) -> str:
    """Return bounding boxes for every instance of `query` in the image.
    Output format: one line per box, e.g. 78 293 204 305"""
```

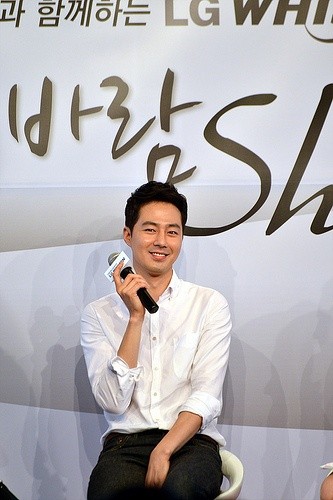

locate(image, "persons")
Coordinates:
79 179 233 500
320 462 333 500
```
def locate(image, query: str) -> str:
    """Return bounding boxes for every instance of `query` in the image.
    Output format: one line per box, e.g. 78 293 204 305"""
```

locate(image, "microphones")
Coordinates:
104 251 159 314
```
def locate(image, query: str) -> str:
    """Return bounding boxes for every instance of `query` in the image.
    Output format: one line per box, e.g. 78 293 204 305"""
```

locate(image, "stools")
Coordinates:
215 446 244 500
